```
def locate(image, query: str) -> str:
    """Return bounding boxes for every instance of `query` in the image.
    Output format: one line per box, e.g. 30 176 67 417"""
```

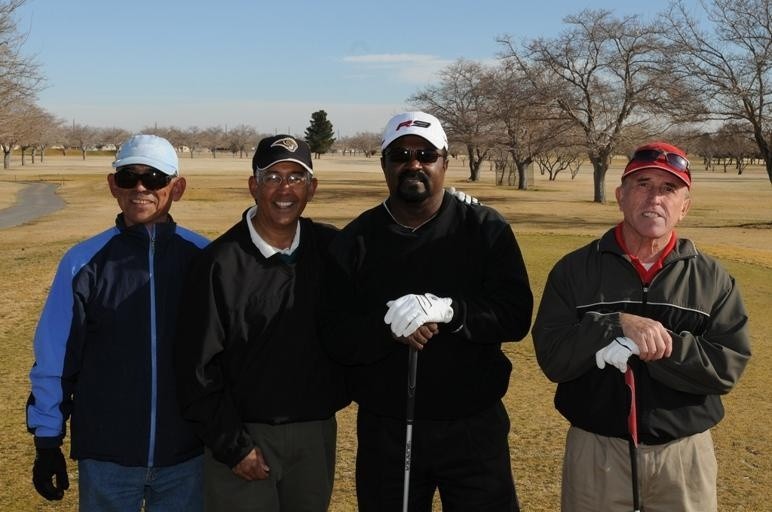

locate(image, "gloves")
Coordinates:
384 293 453 337
596 336 641 374
32 436 69 500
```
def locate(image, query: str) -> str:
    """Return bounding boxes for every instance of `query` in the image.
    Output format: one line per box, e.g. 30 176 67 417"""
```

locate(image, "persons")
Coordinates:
24 134 215 510
168 133 484 512
314 111 535 510
529 141 756 512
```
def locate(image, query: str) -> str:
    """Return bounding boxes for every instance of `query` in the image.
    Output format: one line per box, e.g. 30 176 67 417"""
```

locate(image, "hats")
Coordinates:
621 143 691 189
112 134 179 177
380 111 449 151
252 134 314 177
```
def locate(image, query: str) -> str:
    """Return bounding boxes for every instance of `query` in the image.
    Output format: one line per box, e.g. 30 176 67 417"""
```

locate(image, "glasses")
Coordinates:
113 171 171 191
625 149 691 180
391 147 444 163
262 173 309 189
